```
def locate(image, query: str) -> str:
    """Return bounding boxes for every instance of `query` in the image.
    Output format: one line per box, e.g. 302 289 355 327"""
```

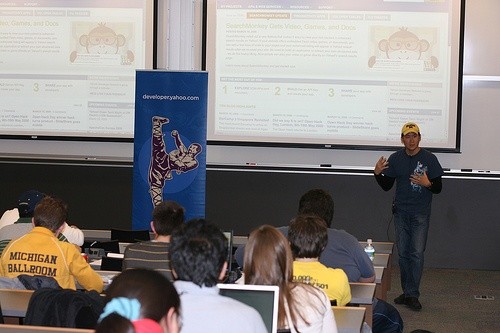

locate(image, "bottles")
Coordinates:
363 238 375 265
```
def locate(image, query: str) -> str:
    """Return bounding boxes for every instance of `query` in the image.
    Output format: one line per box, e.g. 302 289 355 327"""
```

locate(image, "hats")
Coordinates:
17 191 44 215
401 122 420 136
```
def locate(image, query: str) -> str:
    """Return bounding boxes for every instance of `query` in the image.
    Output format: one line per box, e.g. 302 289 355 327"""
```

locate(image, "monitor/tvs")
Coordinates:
218 283 279 333
221 230 233 272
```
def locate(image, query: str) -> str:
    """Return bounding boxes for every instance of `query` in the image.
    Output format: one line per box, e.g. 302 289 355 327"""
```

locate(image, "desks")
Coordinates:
0 229 394 333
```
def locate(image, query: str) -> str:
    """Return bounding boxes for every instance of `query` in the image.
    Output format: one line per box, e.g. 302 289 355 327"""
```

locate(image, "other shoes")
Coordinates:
394 293 405 304
403 296 422 310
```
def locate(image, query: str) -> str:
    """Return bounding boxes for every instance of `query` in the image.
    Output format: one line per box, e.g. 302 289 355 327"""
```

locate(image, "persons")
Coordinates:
122 201 186 283
0 193 84 246
234 188 404 333
288 212 372 333
235 224 338 333
0 191 82 259
373 122 443 312
95 266 181 333
0 197 103 293
168 219 269 333
